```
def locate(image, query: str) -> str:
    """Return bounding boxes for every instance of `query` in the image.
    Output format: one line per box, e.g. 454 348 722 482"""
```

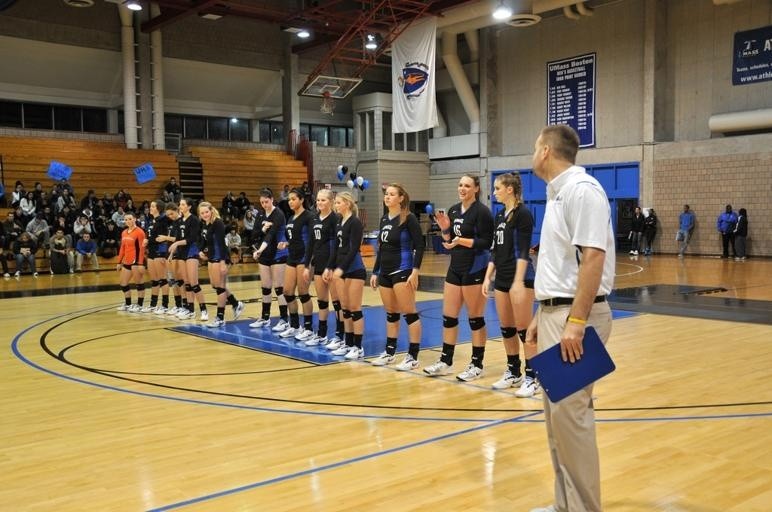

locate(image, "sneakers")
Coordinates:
492 370 524 391
423 360 455 376
249 318 271 328
271 319 364 360
233 301 245 320
33 271 38 277
2 272 10 277
70 267 74 274
720 253 747 260
678 253 683 257
514 375 541 400
629 249 652 256
456 361 485 382
14 271 20 277
371 350 396 365
48 267 54 275
206 317 225 328
116 304 208 321
395 353 420 371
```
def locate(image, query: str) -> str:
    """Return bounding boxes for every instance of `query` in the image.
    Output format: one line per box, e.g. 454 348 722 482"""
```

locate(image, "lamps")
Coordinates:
493 1 511 18
365 33 378 50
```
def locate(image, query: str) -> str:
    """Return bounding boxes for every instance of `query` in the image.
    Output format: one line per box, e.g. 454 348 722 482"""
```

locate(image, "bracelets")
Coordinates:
566 315 587 325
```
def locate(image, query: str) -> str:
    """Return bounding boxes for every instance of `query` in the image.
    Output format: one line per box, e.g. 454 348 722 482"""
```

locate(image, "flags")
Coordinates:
388 15 439 137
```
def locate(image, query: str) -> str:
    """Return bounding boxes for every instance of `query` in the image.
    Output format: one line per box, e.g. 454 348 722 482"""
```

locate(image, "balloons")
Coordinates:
425 204 433 214
335 163 369 194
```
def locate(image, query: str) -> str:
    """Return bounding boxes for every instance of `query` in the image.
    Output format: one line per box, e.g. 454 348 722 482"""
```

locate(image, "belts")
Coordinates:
540 294 606 308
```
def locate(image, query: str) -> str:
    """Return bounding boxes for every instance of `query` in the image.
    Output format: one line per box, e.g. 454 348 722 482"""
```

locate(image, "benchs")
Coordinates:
182 145 308 209
0 135 180 274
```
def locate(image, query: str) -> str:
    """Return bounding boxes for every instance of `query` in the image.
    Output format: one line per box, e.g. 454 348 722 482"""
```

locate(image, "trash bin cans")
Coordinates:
363 235 377 255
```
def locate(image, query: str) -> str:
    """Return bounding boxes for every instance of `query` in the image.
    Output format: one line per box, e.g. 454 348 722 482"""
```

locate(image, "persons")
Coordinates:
481 170 543 398
302 188 345 350
628 206 645 256
117 280 315 341
1 175 182 279
716 203 737 261
195 190 264 264
277 180 316 211
674 204 696 258
321 191 365 360
522 120 618 511
369 182 426 371
731 208 748 261
422 172 495 383
640 208 657 256
